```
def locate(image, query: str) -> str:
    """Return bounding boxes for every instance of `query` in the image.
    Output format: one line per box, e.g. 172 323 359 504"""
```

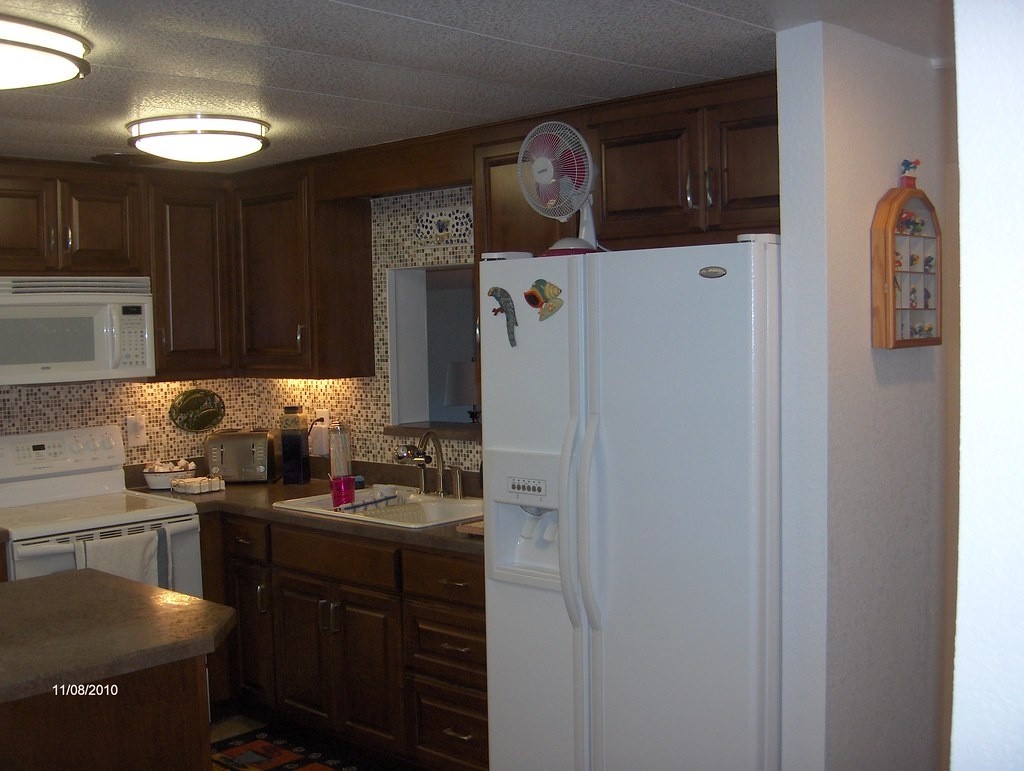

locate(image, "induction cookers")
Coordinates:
1 424 198 541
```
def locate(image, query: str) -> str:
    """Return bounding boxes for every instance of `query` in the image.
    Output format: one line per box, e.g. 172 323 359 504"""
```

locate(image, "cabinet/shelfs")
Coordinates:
581 69 781 252
0 156 143 275
223 514 275 714
270 521 404 762
234 158 375 379
115 168 234 383
870 188 942 350
472 107 580 405
0 654 213 771
402 544 490 771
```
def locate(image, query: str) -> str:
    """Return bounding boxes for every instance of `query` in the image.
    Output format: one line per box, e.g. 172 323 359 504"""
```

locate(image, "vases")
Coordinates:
900 176 916 188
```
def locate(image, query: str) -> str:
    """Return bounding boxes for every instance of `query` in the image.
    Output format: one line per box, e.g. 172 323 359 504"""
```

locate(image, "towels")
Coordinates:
84 531 159 587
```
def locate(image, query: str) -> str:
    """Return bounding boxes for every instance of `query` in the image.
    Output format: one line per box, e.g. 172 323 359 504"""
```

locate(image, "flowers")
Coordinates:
900 159 921 176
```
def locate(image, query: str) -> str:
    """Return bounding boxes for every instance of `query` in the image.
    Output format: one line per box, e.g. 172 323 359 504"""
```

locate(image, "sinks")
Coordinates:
362 501 484 528
294 490 425 515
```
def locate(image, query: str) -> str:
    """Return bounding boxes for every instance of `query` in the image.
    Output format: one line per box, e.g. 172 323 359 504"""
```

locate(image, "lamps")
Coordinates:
0 13 92 92
124 114 272 162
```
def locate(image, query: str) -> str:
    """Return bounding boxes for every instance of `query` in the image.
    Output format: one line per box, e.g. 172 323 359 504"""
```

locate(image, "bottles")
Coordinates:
280 406 310 486
328 421 352 477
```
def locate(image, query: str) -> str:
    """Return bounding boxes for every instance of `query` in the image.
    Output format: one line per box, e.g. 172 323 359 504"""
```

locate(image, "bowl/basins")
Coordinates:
142 469 196 489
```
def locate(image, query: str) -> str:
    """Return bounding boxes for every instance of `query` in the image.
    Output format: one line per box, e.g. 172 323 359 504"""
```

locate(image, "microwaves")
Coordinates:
0 277 155 384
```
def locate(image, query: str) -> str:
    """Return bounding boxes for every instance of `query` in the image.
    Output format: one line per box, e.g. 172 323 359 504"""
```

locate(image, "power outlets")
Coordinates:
315 409 328 429
127 415 146 448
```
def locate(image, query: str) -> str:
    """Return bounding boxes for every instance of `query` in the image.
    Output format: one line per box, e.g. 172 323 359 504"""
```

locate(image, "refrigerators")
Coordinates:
480 235 778 770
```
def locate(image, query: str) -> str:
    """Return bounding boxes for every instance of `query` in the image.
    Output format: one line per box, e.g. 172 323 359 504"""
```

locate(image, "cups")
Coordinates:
331 477 356 512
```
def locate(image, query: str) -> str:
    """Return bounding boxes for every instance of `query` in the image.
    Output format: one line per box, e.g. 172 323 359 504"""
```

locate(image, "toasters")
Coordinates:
204 428 281 482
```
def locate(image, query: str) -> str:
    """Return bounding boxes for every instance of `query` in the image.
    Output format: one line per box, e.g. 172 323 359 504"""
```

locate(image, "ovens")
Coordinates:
12 515 211 722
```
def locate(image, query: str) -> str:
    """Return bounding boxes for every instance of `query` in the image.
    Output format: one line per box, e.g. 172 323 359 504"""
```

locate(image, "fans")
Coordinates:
517 121 598 257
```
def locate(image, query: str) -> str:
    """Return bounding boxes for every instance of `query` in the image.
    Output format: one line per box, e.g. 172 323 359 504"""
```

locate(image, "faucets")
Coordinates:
393 430 448 497
